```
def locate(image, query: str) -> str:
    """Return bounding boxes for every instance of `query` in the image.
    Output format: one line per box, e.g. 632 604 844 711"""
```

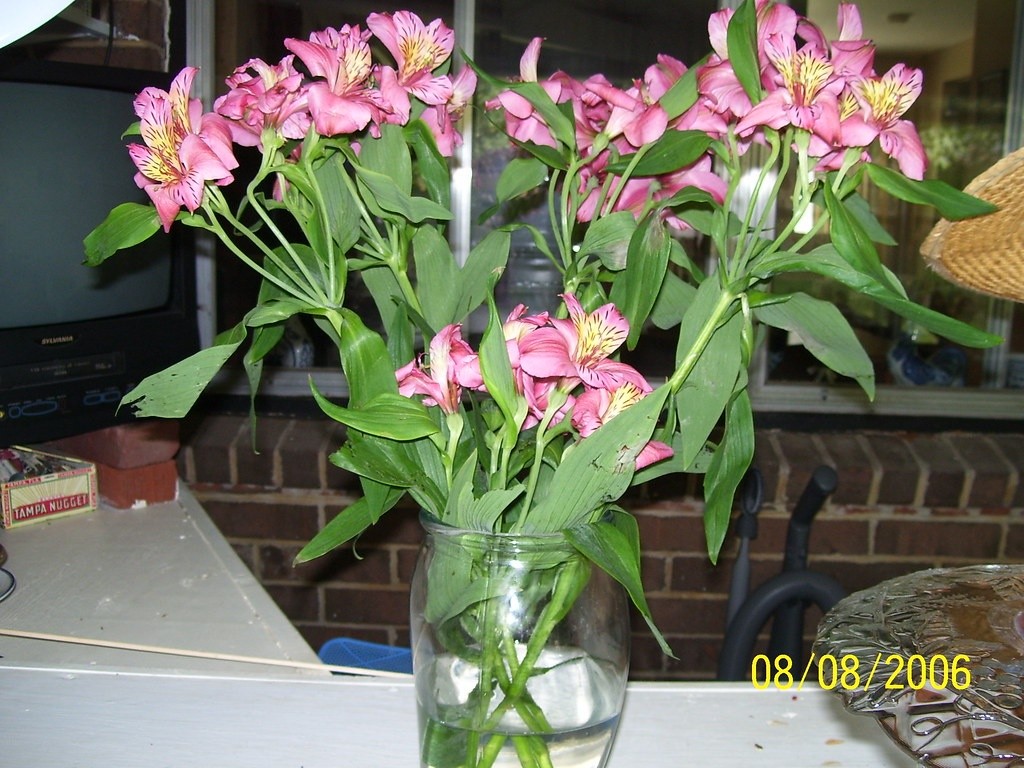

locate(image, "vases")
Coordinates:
409 509 632 768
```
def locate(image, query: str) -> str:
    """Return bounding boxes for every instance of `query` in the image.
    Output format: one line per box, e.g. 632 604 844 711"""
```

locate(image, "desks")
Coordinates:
0 473 925 766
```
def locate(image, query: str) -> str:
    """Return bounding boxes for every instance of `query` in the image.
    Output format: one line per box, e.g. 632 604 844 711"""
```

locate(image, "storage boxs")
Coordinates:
0 445 99 530
43 417 180 510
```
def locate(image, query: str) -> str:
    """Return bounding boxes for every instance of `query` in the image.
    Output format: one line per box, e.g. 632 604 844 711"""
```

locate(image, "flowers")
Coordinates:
83 0 1002 768
